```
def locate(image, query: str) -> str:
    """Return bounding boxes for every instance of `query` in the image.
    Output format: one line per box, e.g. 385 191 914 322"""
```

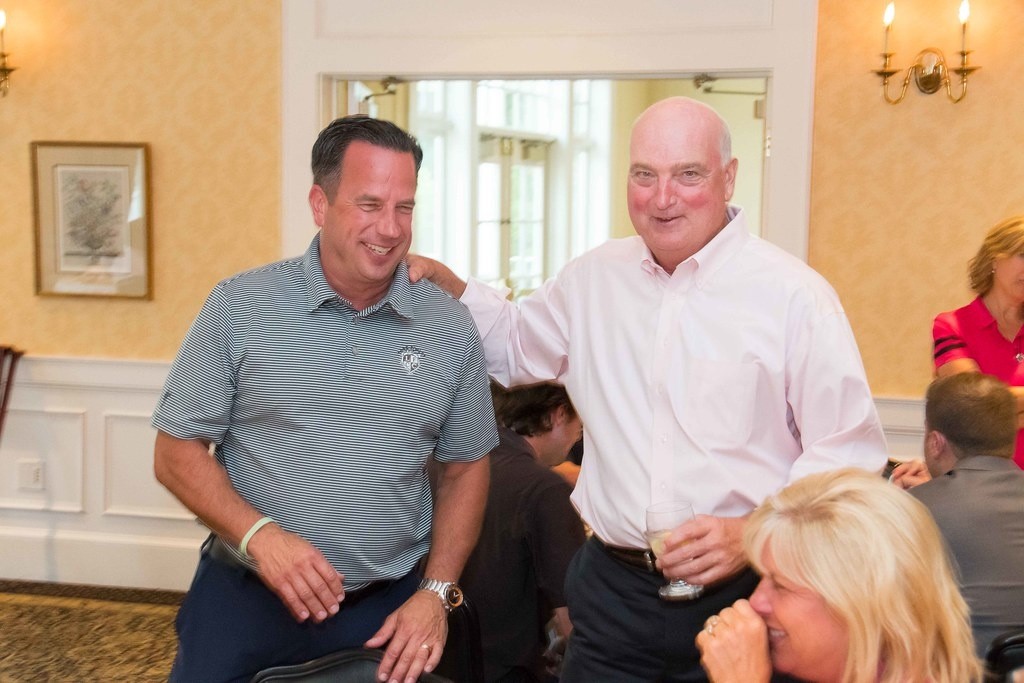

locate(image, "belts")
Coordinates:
592 532 656 570
203 534 247 576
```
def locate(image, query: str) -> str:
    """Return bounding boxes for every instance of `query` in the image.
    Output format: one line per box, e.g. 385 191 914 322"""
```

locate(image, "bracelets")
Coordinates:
240 516 278 561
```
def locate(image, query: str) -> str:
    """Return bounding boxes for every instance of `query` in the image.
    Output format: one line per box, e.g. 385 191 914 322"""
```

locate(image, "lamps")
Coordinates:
870 0 982 105
0 10 17 98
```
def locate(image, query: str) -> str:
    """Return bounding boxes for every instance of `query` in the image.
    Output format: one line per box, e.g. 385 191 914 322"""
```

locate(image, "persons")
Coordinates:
932 217 1024 468
413 381 589 683
890 370 1024 663
149 115 500 683
694 467 981 683
403 97 888 683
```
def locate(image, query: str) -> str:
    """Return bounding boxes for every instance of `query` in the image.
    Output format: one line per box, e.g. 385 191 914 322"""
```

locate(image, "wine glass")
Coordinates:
648 504 702 598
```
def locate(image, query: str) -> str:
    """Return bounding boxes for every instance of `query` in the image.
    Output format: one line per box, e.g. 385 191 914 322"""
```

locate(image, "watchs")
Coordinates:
416 578 465 612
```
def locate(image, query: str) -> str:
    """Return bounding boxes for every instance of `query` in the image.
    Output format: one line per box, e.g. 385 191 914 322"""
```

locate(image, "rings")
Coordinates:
421 642 432 654
708 618 722 633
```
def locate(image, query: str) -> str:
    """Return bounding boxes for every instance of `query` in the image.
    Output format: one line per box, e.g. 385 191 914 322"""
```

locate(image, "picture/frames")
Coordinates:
30 141 154 302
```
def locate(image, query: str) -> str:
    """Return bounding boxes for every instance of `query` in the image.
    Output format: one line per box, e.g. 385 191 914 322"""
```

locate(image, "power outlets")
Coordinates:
16 458 46 490
